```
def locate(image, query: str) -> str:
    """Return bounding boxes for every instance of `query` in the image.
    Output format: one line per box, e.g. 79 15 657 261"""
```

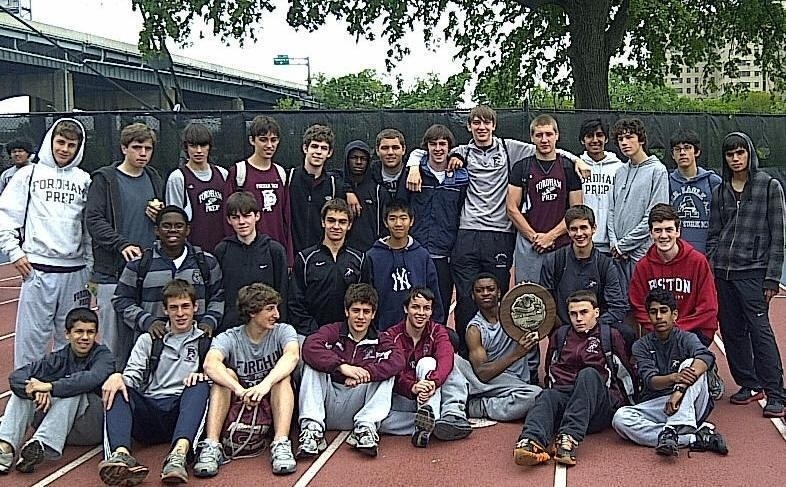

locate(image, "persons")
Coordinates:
0 106 786 476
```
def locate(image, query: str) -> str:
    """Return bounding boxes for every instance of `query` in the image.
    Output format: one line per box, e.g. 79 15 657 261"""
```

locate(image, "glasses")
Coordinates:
160 224 185 231
674 145 691 153
